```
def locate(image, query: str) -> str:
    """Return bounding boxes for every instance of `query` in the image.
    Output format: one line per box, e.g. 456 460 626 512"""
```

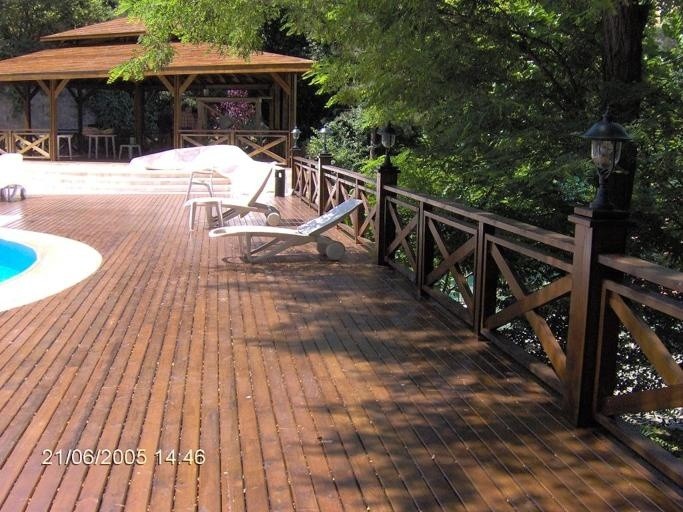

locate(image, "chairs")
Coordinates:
209 197 364 264
186 161 281 231
0 152 26 203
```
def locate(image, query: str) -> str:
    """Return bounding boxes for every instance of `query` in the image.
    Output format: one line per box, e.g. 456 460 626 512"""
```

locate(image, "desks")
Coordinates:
88 134 117 159
40 134 75 157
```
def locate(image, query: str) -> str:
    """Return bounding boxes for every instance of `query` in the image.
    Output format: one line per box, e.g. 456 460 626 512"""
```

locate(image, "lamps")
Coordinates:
580 105 632 211
376 120 399 167
318 124 332 153
290 126 302 149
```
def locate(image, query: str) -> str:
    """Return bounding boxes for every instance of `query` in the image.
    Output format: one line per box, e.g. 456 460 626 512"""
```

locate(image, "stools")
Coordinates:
118 144 142 161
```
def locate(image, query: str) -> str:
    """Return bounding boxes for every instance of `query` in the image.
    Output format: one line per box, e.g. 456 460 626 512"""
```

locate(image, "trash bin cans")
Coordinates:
275 169 285 197
